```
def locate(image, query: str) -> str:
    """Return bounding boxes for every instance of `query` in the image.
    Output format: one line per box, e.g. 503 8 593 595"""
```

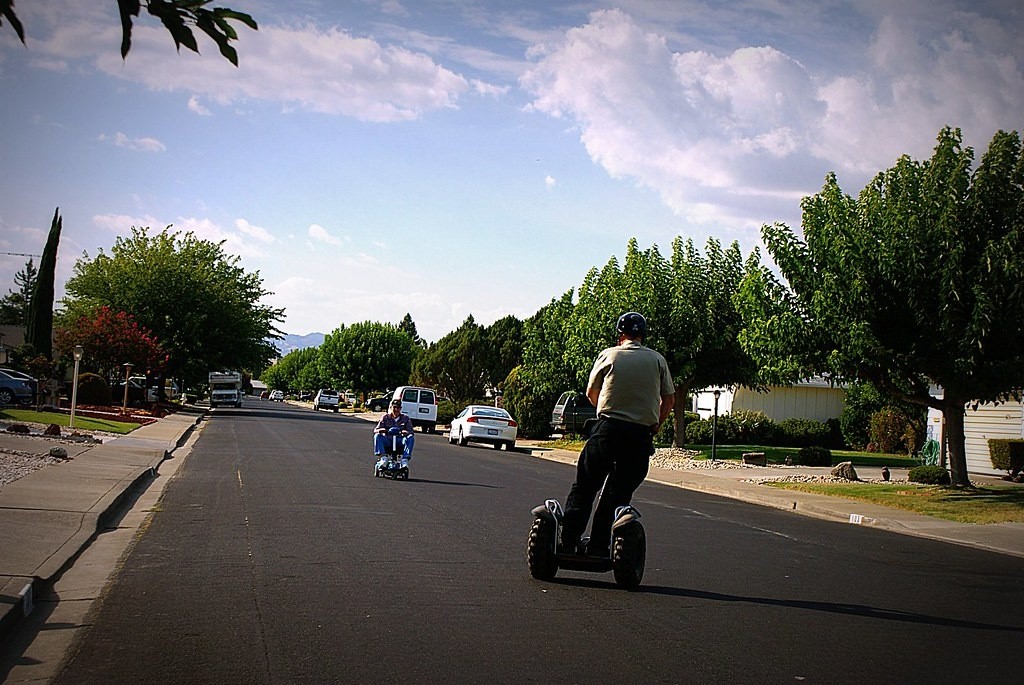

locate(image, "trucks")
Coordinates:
207 370 246 409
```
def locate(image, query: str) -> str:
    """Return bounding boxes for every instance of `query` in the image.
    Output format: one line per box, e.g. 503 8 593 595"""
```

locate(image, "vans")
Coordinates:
389 385 438 436
345 393 356 406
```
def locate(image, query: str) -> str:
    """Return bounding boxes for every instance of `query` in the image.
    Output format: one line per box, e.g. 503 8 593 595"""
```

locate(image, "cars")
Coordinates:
259 391 270 400
449 404 518 450
0 371 33 407
0 368 41 406
301 392 314 402
364 390 394 412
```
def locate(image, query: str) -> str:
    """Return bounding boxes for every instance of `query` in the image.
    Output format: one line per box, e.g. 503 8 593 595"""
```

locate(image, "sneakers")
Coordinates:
402 459 409 469
380 456 388 468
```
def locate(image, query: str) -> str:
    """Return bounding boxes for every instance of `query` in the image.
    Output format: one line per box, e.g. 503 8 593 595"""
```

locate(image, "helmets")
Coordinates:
616 311 647 337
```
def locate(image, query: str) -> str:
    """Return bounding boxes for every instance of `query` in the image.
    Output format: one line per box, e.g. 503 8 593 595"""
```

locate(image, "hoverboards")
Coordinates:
526 418 649 591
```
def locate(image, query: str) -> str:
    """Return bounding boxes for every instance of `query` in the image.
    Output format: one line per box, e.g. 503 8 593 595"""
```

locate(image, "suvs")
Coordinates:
269 390 283 402
550 390 599 436
111 375 179 404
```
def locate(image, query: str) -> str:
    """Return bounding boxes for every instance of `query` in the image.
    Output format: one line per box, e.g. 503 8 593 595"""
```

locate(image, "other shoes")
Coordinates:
584 541 607 559
557 526 577 556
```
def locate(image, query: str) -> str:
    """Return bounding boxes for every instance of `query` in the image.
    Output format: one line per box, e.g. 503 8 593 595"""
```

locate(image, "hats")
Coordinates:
392 400 402 408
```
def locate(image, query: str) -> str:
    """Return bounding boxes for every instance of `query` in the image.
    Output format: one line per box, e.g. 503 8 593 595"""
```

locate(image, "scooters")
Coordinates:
375 426 412 481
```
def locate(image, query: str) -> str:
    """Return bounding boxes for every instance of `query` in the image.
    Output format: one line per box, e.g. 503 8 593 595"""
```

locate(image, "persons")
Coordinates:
372 400 415 471
557 309 676 559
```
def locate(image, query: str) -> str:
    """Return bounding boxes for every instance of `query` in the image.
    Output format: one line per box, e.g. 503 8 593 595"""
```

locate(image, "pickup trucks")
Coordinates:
314 389 339 413
